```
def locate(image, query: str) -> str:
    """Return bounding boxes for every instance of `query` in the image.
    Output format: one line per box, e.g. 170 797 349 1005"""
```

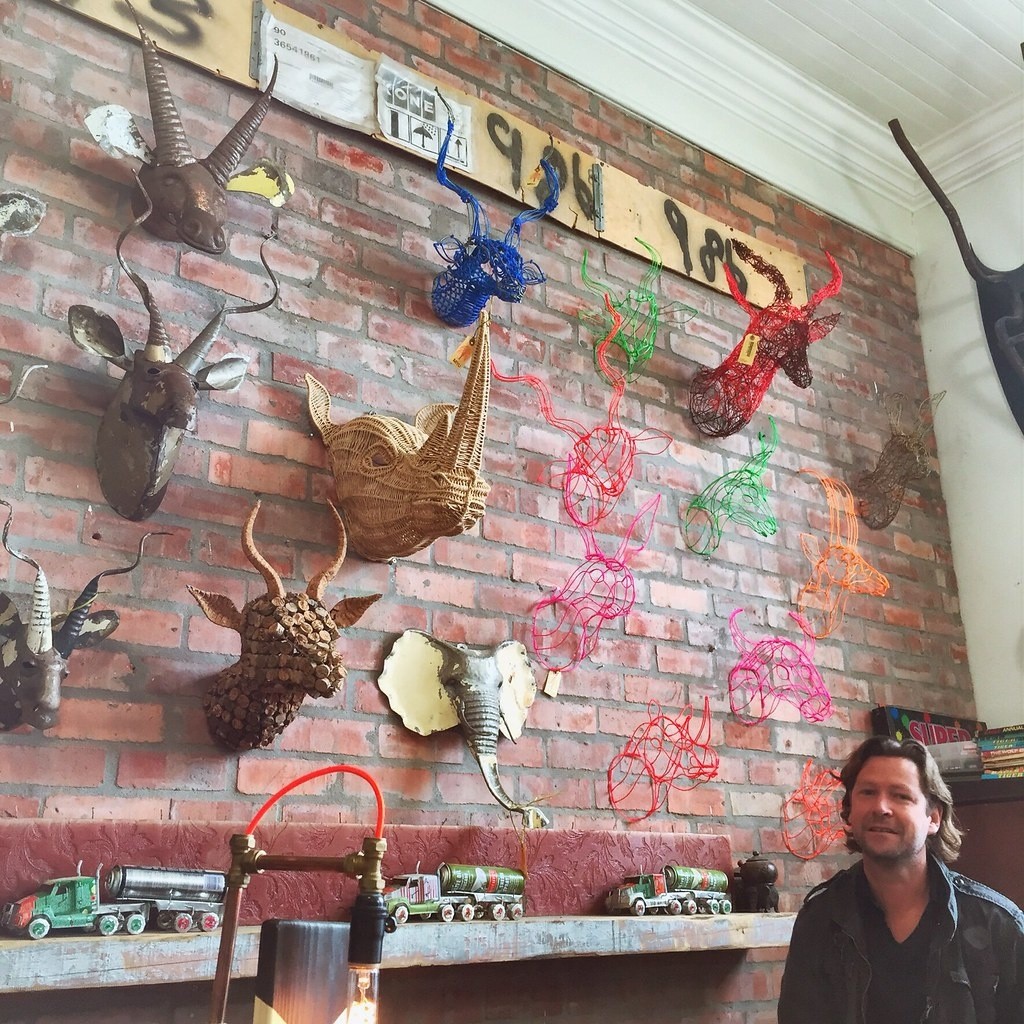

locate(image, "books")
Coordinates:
975 724 1024 779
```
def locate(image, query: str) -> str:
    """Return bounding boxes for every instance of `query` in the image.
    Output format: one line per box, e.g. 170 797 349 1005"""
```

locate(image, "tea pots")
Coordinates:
737 850 778 886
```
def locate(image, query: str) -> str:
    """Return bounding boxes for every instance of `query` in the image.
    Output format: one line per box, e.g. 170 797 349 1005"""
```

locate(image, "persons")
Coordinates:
776 739 1024 1024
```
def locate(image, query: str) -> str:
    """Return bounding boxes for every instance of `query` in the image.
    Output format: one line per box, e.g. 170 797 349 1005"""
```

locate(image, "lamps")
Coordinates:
335 892 389 1024
